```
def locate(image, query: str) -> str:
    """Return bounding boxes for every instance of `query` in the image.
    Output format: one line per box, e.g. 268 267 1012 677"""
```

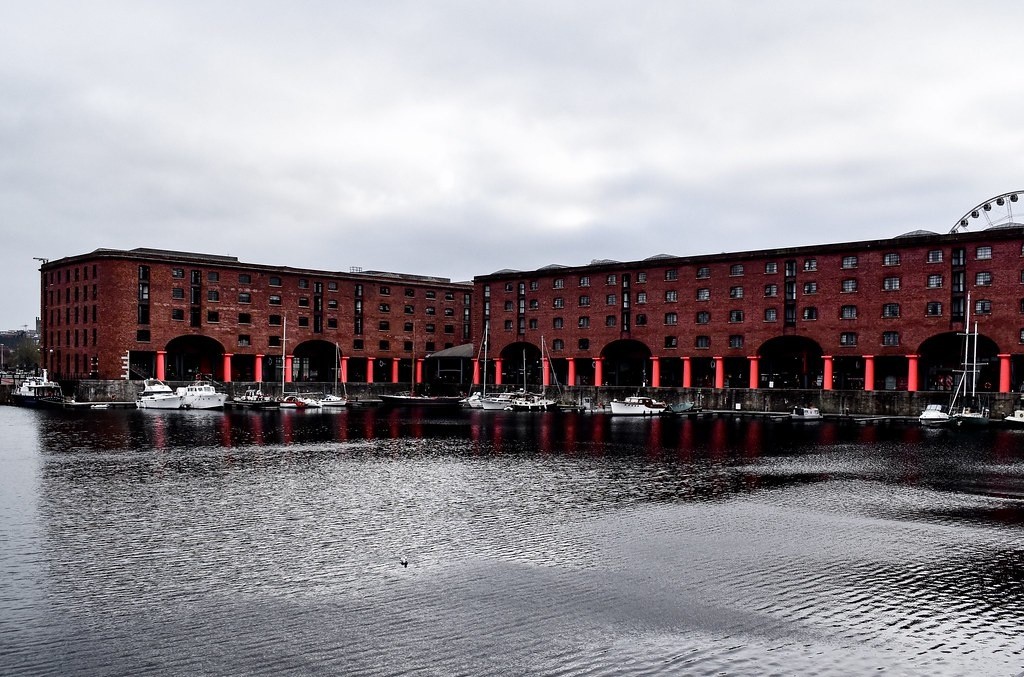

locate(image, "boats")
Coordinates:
789 407 824 420
90 403 110 408
233 386 275 406
610 396 667 416
10 368 66 410
921 403 950 427
91 408 108 410
1003 410 1024 424
135 376 229 409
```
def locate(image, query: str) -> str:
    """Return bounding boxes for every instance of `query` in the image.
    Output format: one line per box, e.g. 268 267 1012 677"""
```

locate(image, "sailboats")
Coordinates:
279 316 347 408
457 321 566 412
377 320 466 403
946 290 991 424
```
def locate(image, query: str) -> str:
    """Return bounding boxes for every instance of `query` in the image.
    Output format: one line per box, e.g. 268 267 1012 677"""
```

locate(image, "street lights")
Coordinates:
50 348 54 382
